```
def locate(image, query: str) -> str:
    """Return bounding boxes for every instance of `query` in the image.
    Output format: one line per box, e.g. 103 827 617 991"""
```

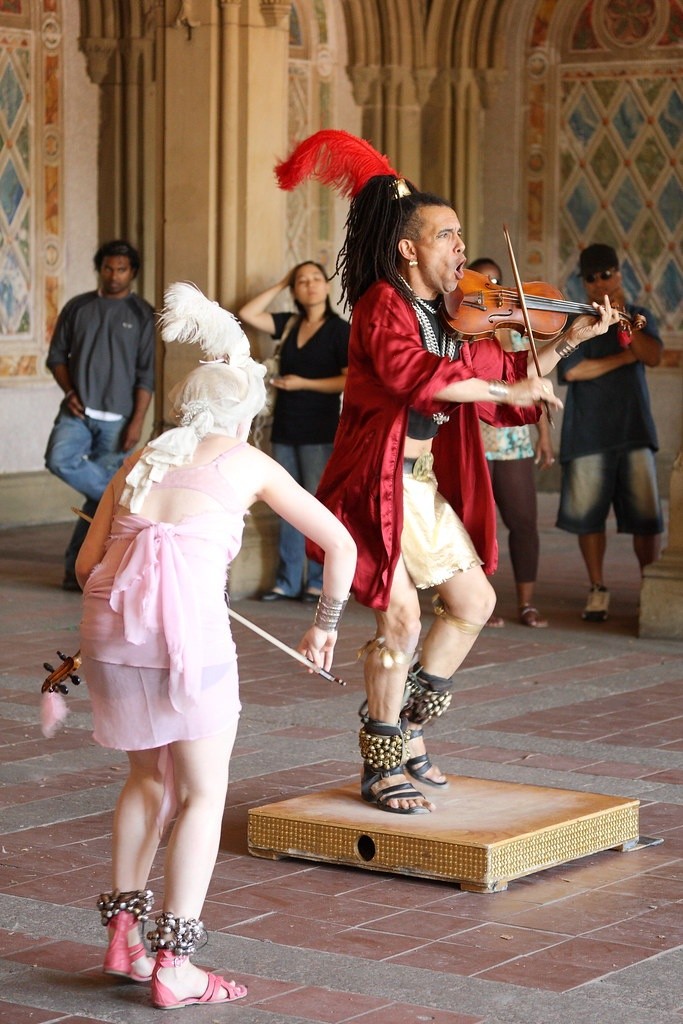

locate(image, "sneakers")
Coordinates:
582 585 611 622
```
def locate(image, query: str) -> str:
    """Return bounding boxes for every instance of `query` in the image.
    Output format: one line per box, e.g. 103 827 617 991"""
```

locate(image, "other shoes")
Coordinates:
301 589 320 603
260 589 300 601
62 575 83 592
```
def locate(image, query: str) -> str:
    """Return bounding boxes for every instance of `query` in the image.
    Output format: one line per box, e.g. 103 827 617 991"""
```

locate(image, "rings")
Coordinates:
543 385 547 391
552 458 555 463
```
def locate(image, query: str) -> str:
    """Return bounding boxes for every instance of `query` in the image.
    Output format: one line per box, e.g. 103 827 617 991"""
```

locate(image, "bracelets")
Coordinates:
488 379 508 405
553 331 578 358
314 592 350 632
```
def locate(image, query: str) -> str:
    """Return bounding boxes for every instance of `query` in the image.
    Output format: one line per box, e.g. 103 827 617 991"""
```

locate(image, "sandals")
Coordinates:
519 604 548 629
484 614 504 629
151 953 246 1009
400 720 454 788
103 911 157 980
361 767 437 815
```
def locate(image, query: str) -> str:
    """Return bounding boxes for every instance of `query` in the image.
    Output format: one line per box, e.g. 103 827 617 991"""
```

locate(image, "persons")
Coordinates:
43 241 155 592
554 244 665 621
238 261 351 603
468 257 555 628
306 172 622 815
75 365 358 1010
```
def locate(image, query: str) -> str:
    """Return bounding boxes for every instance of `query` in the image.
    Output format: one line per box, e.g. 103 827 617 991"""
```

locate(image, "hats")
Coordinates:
576 243 617 276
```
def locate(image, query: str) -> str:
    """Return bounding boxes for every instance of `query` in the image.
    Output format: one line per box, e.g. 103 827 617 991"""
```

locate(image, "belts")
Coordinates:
401 451 434 481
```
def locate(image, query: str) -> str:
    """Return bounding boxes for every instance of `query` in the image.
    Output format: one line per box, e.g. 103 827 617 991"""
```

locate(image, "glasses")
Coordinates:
584 268 619 283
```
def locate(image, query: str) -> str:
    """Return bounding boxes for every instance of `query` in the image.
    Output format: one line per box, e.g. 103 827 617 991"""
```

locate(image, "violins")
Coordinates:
440 268 646 342
40 646 86 697
